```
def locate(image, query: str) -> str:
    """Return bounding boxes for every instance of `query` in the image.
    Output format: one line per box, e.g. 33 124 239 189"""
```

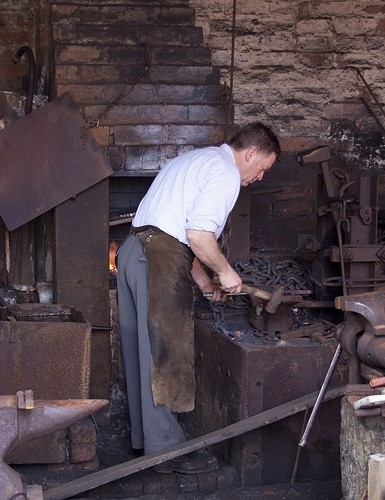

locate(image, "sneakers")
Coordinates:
153 452 219 474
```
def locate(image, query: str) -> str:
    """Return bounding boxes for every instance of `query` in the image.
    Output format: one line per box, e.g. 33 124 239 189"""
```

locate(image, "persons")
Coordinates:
115 122 283 475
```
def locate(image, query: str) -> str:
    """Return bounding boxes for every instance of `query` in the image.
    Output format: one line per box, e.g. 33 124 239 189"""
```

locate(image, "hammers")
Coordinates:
239 281 305 315
